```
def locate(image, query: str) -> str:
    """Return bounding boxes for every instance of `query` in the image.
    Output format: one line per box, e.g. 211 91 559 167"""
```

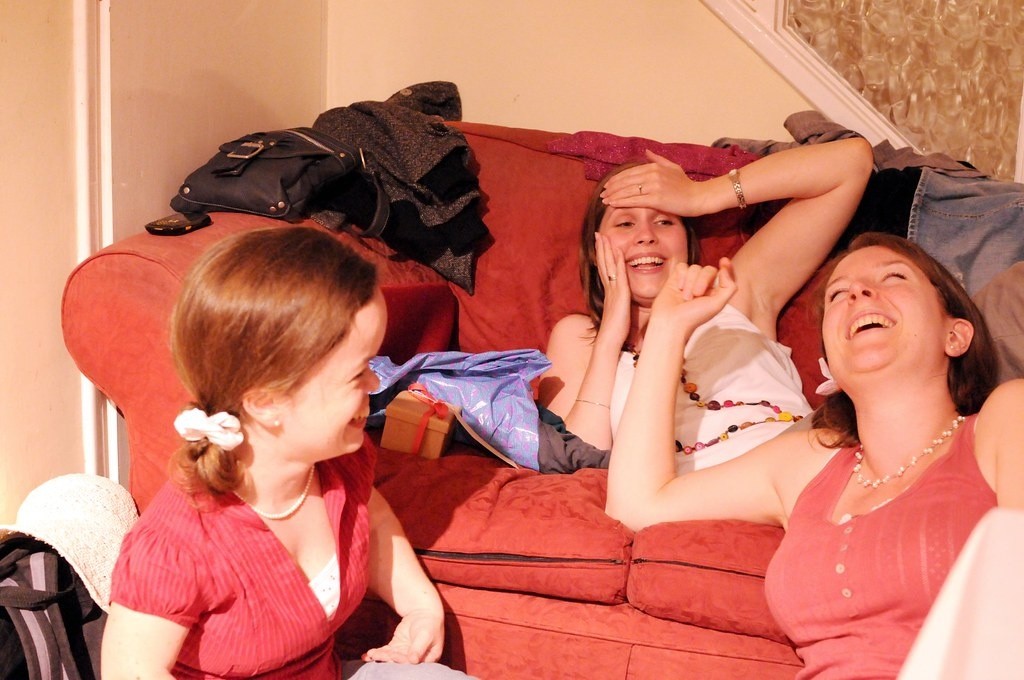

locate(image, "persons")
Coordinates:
539 137 873 479
606 232 1024 680
101 226 476 679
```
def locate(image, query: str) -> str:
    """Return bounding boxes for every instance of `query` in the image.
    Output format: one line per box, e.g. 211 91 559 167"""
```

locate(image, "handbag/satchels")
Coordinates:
170 127 391 239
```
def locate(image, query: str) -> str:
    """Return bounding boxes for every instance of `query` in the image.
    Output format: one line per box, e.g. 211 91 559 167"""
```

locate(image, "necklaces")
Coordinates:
631 339 803 454
854 414 967 489
235 465 315 519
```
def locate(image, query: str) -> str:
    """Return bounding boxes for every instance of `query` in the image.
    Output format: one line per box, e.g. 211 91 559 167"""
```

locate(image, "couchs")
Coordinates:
63 122 1024 680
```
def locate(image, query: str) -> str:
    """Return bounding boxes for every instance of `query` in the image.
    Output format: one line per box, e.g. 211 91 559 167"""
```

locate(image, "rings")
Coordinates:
638 184 643 192
609 276 616 281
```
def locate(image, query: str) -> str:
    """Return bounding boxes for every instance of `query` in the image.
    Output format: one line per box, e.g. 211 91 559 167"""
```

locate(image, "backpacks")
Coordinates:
0 527 110 680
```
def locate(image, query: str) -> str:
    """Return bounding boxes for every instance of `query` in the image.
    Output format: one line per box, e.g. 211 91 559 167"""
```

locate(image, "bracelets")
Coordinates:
729 168 746 210
575 399 610 409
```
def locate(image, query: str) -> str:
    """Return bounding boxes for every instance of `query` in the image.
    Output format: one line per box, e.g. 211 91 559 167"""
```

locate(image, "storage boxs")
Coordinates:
380 390 463 459
